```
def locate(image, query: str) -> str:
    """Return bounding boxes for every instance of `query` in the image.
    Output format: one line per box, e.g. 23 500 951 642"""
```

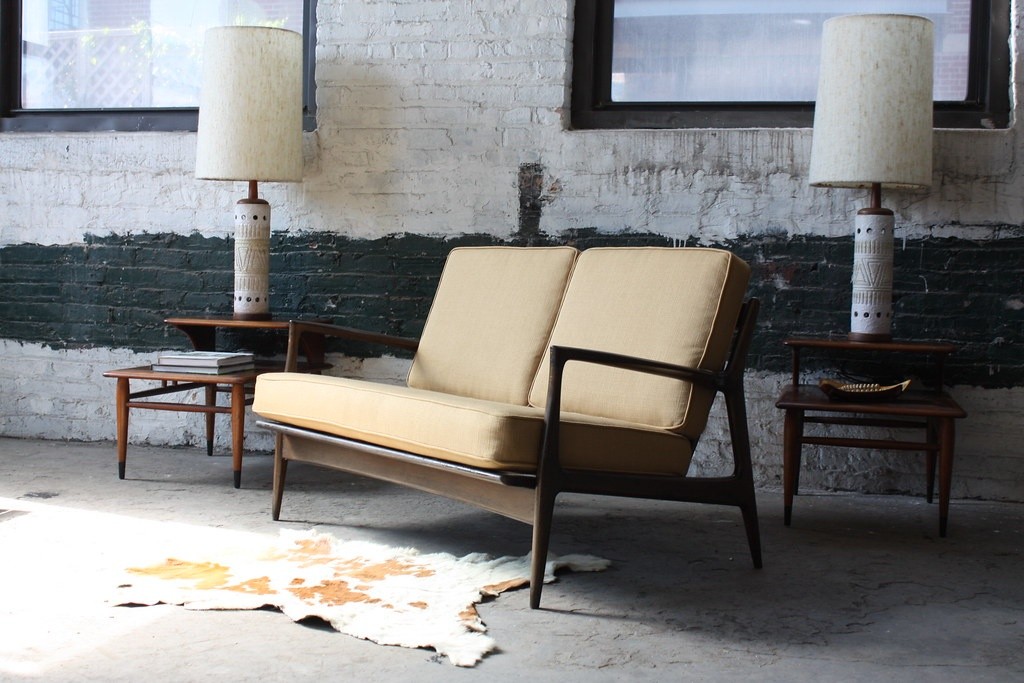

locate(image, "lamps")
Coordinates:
197 26 302 319
810 11 934 333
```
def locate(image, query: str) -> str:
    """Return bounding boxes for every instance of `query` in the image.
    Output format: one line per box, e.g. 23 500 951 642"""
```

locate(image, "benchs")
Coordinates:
251 244 761 614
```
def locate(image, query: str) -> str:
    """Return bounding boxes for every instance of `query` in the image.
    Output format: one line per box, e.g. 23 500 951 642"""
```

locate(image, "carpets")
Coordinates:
115 527 608 667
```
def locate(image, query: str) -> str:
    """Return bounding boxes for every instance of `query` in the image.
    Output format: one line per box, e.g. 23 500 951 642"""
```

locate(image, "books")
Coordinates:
151 351 256 375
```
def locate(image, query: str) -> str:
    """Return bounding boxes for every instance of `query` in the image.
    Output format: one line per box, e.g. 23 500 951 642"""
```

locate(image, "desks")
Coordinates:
776 337 968 542
104 317 332 495
